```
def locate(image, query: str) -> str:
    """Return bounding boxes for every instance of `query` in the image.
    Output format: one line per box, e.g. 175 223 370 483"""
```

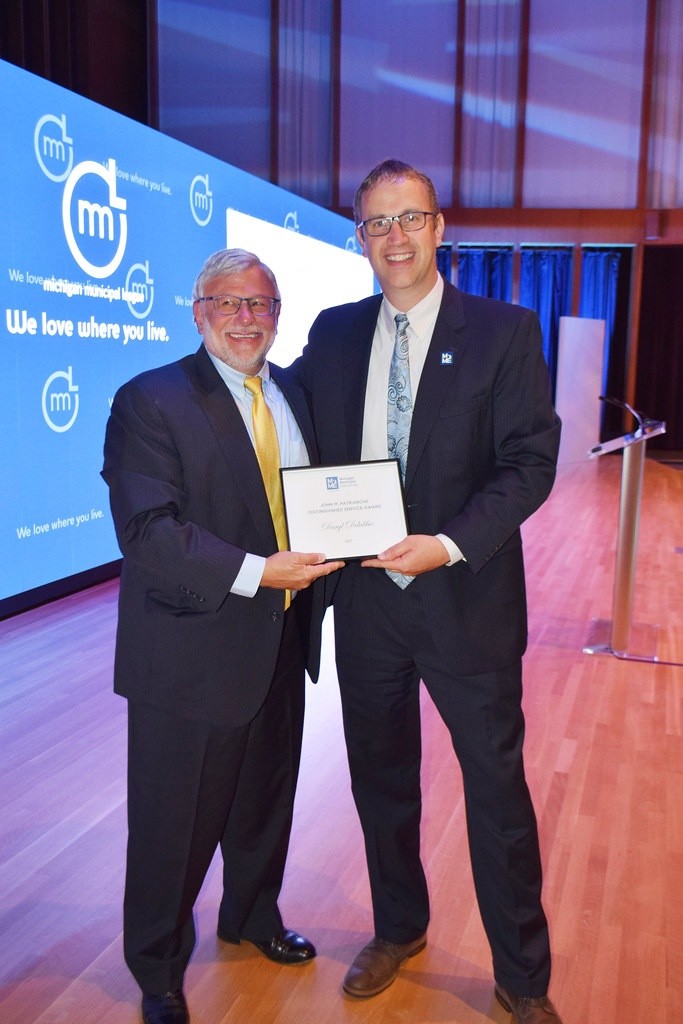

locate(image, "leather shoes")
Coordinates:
217 922 317 965
142 989 189 1024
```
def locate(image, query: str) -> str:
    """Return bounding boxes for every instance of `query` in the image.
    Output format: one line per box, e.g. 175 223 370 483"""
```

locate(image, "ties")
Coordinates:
385 314 416 590
244 376 291 613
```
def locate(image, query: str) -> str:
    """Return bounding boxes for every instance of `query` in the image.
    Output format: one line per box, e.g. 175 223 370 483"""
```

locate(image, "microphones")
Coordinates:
600 394 662 438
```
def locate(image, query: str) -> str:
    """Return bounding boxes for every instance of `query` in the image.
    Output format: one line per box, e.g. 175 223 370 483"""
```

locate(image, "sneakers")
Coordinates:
494 978 563 1024
343 932 427 997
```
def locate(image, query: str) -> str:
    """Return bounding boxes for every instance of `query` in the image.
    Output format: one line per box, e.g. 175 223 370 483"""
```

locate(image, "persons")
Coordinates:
280 159 564 1024
100 249 347 1024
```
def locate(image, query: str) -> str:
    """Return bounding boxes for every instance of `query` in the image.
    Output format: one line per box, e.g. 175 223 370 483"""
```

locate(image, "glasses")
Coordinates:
196 295 280 316
357 212 437 237
